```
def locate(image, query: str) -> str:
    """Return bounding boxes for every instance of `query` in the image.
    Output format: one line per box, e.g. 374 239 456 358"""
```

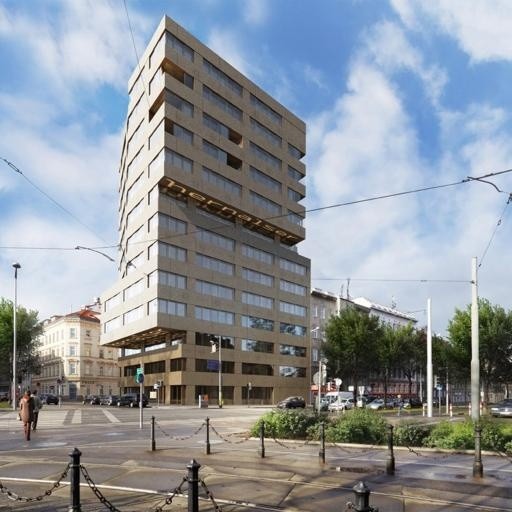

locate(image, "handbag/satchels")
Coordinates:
16 412 22 421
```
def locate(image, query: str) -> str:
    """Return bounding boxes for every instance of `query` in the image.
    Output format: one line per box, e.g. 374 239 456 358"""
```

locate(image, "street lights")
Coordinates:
11 263 21 409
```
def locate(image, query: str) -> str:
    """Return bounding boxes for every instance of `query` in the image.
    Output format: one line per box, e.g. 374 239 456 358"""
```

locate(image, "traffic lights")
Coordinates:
327 367 331 382
331 380 335 389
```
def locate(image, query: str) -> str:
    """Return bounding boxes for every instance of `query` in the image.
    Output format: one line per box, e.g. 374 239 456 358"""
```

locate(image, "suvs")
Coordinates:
276 395 306 410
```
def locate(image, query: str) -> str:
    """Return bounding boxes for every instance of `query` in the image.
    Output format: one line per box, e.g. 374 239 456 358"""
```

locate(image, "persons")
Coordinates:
29 390 42 430
19 390 35 441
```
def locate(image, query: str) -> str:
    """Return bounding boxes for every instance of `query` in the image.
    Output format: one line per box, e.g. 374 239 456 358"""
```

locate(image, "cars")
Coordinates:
82 393 147 408
40 393 58 406
318 390 451 411
487 398 512 419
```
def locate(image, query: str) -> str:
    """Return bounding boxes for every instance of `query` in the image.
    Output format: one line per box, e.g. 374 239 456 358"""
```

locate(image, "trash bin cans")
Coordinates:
199 394 208 409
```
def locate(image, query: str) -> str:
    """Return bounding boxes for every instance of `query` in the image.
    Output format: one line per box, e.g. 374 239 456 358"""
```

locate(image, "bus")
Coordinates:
1 385 9 401
